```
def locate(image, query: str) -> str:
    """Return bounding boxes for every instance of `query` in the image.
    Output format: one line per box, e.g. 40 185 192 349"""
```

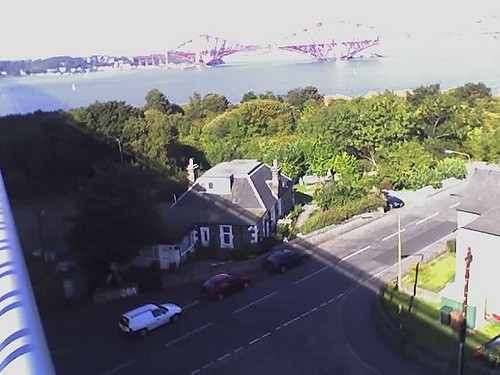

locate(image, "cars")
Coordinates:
263 247 308 274
199 273 251 301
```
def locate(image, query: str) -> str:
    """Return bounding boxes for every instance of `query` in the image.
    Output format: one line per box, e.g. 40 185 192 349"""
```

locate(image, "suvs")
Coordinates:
118 303 183 337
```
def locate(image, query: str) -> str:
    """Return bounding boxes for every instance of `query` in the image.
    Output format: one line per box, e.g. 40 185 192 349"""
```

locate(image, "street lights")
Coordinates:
445 150 471 164
360 211 402 292
